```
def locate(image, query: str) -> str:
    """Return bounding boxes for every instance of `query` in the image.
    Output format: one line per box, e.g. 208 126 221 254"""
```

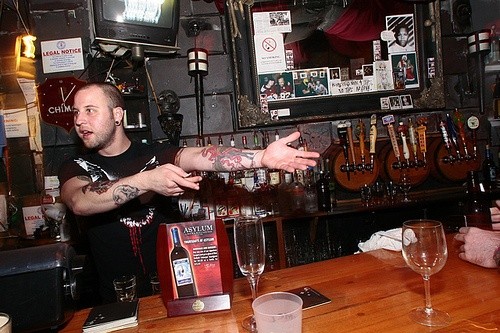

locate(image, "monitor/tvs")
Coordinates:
93 0 181 46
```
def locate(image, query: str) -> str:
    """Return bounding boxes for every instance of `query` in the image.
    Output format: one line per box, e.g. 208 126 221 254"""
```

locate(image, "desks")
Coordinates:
51 232 500 333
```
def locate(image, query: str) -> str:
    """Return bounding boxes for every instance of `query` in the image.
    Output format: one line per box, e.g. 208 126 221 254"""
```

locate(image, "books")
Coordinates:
83 299 141 333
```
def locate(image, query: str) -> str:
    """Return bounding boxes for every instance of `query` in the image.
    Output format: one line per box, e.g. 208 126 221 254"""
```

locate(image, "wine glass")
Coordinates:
402 220 450 326
234 216 265 331
360 173 412 208
40 190 70 242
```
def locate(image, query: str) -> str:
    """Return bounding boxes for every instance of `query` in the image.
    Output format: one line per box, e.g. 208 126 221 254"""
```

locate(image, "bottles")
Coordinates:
482 145 496 181
183 130 336 219
461 171 493 231
170 227 197 299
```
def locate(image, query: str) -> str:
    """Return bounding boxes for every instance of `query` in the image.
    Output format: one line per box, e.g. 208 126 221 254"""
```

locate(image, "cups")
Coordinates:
113 275 136 302
252 292 303 333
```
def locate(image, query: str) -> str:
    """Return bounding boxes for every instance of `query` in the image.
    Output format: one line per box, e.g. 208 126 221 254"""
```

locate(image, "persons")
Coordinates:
59 83 320 310
390 24 415 81
453 200 500 268
260 76 328 98
